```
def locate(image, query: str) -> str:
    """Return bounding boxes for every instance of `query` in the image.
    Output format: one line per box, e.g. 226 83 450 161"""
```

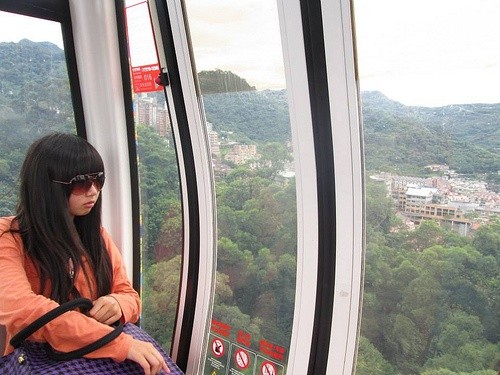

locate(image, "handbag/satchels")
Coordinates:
0 297 184 375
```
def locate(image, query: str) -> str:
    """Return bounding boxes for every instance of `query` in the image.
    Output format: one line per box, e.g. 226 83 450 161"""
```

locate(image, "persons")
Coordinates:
0 132 171 375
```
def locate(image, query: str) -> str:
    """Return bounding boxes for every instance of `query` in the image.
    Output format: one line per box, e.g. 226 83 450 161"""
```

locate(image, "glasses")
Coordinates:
50 172 106 196
